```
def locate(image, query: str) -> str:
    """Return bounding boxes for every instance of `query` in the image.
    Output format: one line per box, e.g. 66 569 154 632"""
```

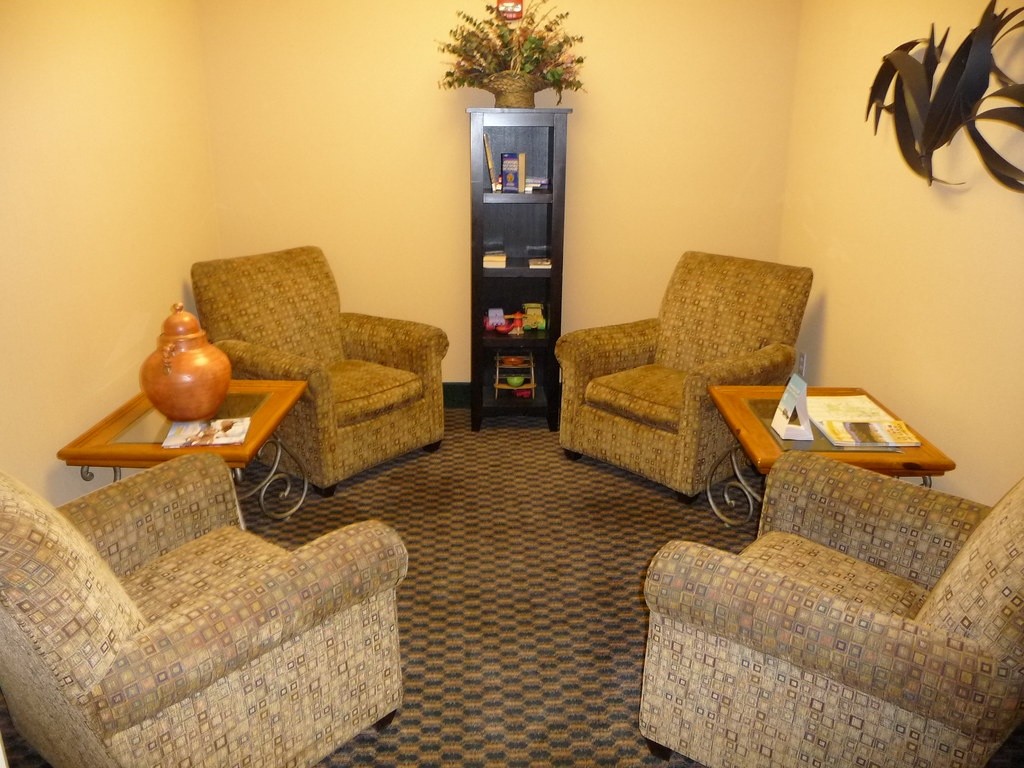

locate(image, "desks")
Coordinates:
57 380 308 531
707 386 956 526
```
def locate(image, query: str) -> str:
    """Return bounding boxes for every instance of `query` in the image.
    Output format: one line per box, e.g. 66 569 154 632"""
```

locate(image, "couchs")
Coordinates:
639 450 1024 768
555 251 813 503
0 453 408 768
190 247 449 498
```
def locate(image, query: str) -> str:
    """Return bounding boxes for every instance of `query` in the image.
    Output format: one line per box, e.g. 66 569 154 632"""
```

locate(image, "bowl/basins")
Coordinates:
507 376 524 387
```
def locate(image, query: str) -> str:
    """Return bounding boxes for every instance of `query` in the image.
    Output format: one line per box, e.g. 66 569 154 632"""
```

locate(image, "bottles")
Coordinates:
139 303 233 422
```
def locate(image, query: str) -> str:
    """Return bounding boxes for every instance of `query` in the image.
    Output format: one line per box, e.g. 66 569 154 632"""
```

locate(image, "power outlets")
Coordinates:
799 352 806 376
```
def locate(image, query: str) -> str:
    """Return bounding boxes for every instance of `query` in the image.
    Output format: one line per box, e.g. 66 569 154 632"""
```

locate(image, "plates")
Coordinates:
501 357 525 366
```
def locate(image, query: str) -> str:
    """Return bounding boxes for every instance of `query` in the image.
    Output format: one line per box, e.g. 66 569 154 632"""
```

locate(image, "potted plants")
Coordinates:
436 0 589 108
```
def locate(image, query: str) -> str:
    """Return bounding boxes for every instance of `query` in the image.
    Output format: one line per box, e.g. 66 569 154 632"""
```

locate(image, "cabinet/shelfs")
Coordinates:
464 108 573 432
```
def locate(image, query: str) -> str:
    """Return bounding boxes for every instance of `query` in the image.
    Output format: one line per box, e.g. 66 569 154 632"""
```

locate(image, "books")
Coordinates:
501 152 526 194
496 175 548 191
161 415 252 448
805 394 922 447
528 258 553 269
484 133 496 192
483 250 507 269
